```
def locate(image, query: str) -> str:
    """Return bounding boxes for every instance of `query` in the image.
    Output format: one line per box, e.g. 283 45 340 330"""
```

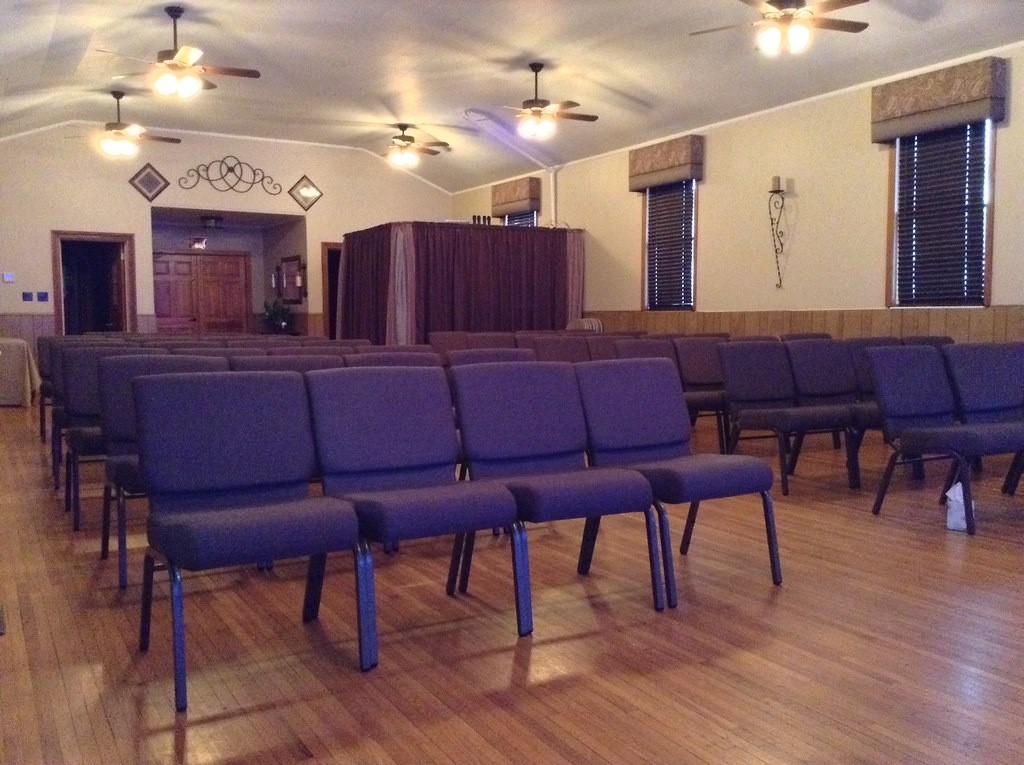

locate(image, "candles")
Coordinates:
772 176 781 190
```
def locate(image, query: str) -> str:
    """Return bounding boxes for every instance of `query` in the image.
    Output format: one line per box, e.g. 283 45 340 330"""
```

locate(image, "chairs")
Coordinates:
34 330 1024 714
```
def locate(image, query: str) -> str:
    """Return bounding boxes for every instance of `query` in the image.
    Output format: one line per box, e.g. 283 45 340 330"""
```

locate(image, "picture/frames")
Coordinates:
289 175 323 212
129 161 171 203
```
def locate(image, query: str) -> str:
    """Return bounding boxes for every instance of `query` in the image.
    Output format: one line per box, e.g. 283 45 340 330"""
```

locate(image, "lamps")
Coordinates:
388 148 421 167
93 131 142 159
753 20 816 57
201 217 223 229
147 64 203 95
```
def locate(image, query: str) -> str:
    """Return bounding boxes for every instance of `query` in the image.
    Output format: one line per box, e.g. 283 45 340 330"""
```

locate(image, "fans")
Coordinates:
743 0 869 33
488 62 598 122
352 124 449 158
66 90 183 143
97 5 260 90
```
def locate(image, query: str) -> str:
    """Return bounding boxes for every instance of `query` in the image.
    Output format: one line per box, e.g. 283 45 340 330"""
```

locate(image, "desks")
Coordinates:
0 338 54 407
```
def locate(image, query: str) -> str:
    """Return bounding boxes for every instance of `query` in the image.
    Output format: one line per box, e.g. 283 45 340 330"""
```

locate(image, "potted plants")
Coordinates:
263 300 293 332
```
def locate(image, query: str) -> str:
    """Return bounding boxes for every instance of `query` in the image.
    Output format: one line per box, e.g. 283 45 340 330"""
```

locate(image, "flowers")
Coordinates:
516 113 558 140
